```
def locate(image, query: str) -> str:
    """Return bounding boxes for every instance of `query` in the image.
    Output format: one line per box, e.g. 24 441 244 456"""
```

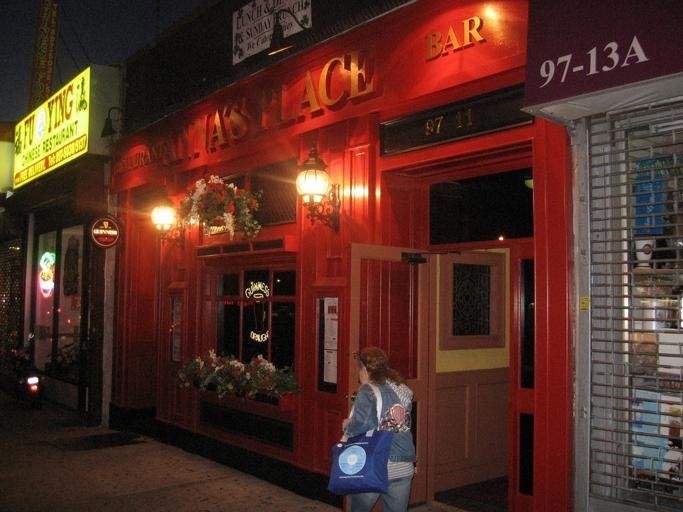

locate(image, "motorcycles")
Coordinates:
6 331 42 410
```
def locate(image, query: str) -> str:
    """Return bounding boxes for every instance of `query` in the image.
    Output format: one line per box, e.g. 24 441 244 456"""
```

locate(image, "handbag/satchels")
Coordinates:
327 381 395 497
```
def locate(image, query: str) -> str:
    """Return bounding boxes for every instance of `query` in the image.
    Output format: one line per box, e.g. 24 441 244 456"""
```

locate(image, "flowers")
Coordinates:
174 347 301 401
176 171 262 241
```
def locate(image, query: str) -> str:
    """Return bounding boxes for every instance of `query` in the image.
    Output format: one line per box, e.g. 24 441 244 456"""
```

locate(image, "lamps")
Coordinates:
267 8 318 56
294 145 341 236
149 189 186 250
100 105 126 138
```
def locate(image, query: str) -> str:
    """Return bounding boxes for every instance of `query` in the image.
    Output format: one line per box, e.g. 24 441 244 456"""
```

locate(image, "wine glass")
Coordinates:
634 240 653 269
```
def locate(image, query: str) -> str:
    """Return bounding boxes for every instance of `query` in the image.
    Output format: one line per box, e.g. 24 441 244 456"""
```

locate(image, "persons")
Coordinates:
340 347 418 512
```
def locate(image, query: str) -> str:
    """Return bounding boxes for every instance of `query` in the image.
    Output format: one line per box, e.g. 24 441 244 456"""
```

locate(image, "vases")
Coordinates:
192 377 298 421
